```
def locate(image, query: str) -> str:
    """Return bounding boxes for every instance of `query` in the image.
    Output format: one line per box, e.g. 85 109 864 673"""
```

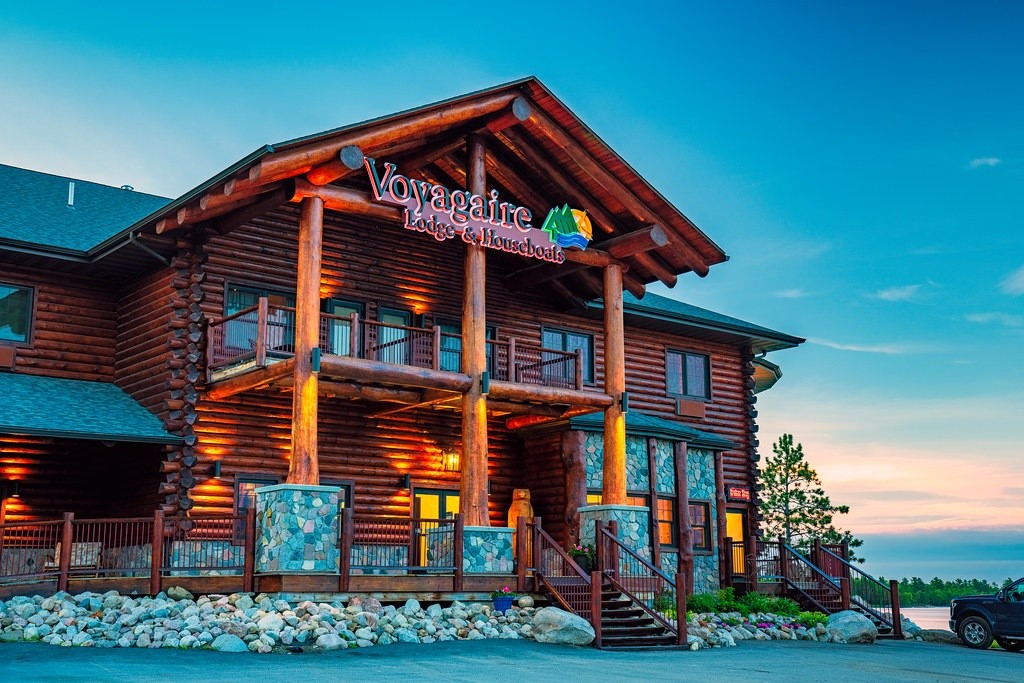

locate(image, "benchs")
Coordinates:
44 543 106 579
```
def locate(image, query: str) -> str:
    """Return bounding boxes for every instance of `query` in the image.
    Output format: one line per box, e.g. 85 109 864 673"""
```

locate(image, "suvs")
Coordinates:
947 576 1024 654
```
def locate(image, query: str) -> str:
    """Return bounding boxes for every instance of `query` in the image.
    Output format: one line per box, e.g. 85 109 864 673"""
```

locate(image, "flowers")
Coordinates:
567 543 596 569
490 586 517 598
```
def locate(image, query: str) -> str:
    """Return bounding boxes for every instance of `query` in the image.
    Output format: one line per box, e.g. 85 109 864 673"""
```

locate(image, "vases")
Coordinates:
493 596 513 615
573 555 590 575
